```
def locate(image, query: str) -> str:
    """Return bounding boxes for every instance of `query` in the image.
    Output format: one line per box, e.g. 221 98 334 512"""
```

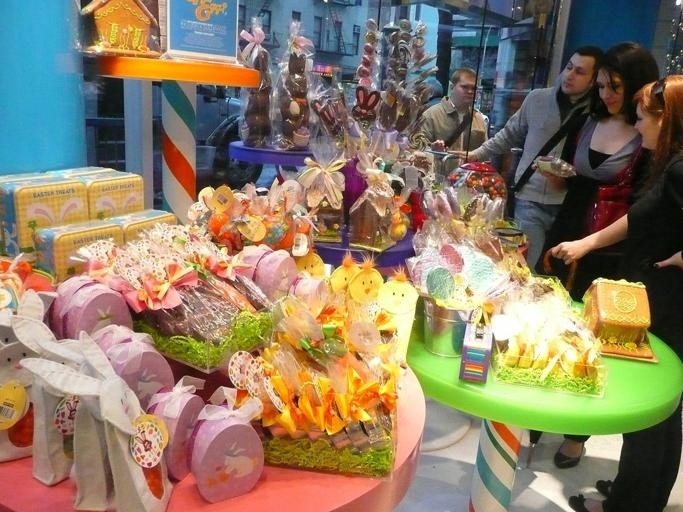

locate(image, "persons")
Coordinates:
567 74 682 511
651 247 681 274
421 67 489 164
526 43 657 467
449 47 602 268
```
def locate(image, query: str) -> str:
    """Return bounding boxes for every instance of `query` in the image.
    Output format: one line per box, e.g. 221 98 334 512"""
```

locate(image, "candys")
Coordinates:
409 237 468 305
209 242 412 452
78 228 275 347
446 172 507 200
183 179 315 255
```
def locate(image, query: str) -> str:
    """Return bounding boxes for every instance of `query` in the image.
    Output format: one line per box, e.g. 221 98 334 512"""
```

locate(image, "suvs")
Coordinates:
83 75 264 193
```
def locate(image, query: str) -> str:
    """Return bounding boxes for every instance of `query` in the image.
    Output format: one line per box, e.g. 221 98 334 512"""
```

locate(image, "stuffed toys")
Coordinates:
278 53 310 152
244 52 271 148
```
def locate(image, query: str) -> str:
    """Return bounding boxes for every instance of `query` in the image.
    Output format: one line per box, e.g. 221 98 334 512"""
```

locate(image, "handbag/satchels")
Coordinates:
585 169 632 233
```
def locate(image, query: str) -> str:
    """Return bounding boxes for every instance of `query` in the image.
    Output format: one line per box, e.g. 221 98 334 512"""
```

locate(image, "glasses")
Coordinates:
652 78 665 107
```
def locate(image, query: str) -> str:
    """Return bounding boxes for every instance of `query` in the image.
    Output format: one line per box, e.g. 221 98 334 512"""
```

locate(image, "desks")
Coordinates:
0 356 429 510
390 298 681 510
228 136 313 169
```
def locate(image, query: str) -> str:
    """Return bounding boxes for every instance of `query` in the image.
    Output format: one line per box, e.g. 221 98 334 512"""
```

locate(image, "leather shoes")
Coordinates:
568 494 588 512
554 439 584 468
595 480 614 497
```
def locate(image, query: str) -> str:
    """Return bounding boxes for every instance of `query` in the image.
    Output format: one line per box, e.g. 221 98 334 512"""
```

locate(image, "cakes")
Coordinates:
486 276 653 377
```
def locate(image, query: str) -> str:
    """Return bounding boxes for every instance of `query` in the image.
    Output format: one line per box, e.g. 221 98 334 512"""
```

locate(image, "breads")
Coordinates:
430 140 446 153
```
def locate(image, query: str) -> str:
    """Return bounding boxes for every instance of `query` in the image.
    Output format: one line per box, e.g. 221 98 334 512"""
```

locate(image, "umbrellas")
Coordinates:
525 247 577 467
